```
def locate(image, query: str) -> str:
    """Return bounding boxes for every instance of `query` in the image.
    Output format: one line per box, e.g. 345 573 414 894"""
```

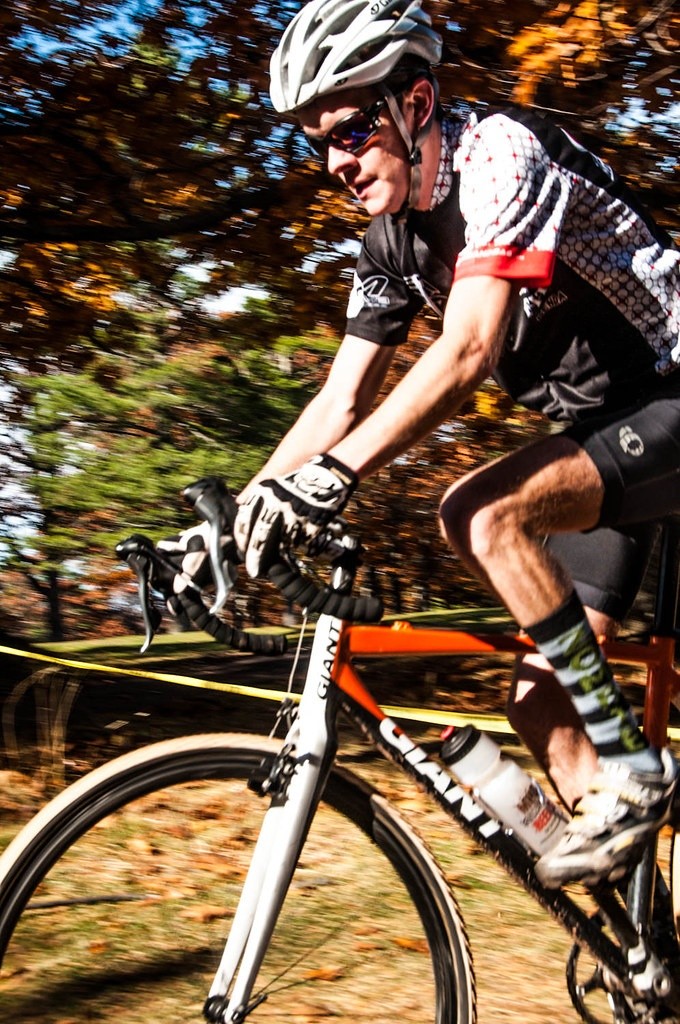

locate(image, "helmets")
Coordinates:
267 0 443 114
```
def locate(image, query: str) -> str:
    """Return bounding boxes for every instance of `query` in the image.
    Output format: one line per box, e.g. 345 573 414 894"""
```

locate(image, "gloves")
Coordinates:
231 452 360 579
150 520 242 601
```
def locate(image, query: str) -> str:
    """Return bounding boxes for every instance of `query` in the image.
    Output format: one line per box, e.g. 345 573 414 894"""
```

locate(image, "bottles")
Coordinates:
438 725 571 857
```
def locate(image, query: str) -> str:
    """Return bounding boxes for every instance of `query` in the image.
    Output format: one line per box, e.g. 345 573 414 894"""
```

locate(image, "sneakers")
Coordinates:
533 747 680 889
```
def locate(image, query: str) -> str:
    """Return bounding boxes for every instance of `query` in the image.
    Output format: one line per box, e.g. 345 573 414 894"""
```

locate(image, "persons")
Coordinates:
147 1 680 887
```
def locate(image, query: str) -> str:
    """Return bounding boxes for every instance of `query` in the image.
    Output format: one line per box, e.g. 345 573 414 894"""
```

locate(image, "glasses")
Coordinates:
303 73 413 160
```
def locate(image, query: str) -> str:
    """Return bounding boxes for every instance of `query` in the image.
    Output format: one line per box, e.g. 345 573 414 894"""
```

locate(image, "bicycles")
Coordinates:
1 469 680 1024
